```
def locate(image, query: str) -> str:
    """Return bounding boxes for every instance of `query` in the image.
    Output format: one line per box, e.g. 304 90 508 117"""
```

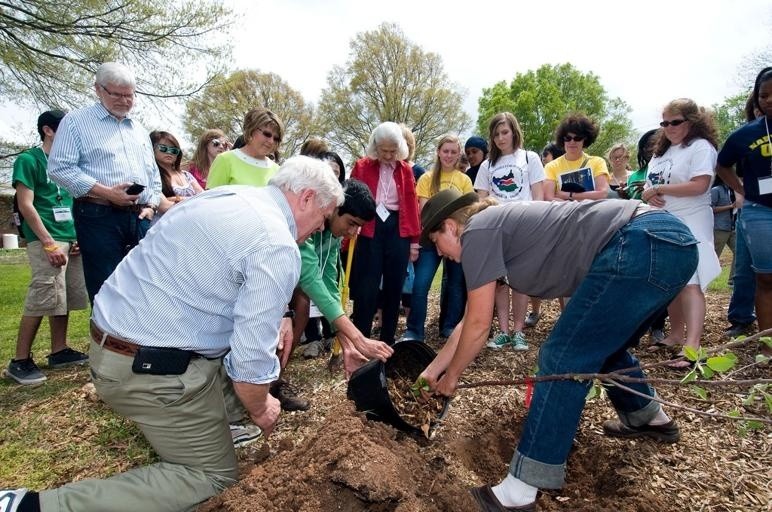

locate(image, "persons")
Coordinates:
416 186 704 509
1 153 348 511
262 178 396 411
47 61 163 308
2 108 94 389
149 63 771 373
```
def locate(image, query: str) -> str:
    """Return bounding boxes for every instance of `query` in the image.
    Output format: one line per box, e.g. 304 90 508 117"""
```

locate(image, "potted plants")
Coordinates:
345 323 772 441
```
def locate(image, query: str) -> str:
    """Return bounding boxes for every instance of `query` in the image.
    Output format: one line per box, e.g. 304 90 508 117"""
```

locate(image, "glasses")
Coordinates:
100 81 135 99
659 117 688 127
562 131 588 144
208 139 228 150
255 125 281 142
155 142 180 157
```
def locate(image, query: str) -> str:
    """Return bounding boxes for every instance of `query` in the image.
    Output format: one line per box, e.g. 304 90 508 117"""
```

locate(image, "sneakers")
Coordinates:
523 313 540 329
511 332 529 352
47 347 88 369
649 330 665 345
486 331 512 351
724 325 752 337
469 483 538 512
0 487 31 512
398 335 417 342
4 355 47 385
228 421 266 452
270 377 312 411
602 417 681 444
302 338 324 358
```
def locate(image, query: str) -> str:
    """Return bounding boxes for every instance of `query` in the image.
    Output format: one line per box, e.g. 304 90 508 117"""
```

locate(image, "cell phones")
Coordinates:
126 182 146 202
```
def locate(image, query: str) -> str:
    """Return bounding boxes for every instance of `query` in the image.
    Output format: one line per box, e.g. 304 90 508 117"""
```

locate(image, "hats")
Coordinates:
417 187 479 252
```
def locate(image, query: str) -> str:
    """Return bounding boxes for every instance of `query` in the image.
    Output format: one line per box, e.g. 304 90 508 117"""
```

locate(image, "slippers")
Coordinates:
667 346 704 371
646 335 685 354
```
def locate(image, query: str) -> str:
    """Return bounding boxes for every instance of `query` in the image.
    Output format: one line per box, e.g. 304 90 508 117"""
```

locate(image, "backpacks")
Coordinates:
11 193 29 239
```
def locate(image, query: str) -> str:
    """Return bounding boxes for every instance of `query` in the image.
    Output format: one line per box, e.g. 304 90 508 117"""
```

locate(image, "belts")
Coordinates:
87 316 210 364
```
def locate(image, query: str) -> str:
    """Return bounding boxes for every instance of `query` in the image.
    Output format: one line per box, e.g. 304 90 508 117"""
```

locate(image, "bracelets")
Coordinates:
42 242 61 254
282 309 296 319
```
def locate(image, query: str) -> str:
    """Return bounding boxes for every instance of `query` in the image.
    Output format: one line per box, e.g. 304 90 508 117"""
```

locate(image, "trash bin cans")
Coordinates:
2 234 20 249
